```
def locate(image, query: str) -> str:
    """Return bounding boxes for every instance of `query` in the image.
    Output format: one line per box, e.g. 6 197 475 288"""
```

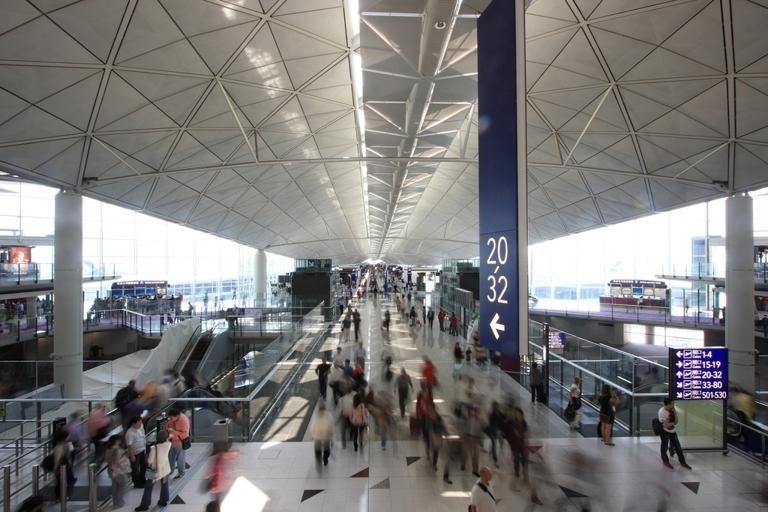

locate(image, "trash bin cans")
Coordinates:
214 418 233 451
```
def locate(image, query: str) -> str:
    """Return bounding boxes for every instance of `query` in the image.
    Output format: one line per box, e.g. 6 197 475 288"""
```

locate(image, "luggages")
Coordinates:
562 400 577 422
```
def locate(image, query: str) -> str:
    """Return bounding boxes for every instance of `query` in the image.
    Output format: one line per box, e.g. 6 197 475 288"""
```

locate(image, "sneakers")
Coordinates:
663 461 673 470
158 500 168 507
134 505 148 512
679 462 691 470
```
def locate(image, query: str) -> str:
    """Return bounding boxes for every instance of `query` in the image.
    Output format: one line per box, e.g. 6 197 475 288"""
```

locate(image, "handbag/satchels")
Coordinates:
39 452 56 473
652 417 664 437
144 465 161 482
567 396 582 410
181 436 192 451
